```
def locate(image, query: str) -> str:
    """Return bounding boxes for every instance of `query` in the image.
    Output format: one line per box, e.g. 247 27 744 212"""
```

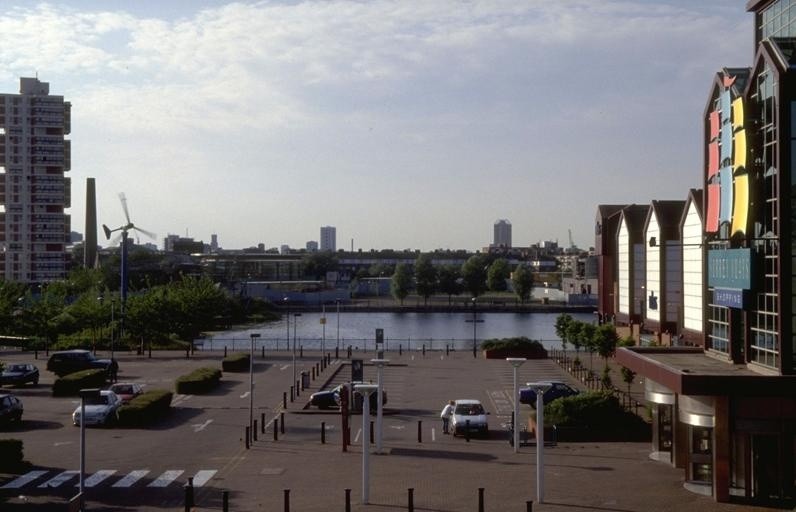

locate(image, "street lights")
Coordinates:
352 384 378 505
506 357 526 453
370 358 389 454
526 382 552 505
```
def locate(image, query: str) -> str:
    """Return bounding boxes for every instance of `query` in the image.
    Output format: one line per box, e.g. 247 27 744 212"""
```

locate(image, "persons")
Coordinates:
439 399 455 436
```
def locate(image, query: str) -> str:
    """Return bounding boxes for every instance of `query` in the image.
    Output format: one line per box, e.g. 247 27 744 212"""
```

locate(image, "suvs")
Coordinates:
309 382 386 409
46 350 118 376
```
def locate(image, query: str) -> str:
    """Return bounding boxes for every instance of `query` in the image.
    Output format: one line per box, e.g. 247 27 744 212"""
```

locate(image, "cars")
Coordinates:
0 394 23 426
71 390 121 429
518 381 579 410
0 363 38 388
449 399 490 436
107 382 143 406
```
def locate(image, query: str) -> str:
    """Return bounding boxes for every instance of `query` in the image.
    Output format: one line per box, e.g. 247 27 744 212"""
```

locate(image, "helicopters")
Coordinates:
102 192 157 244
